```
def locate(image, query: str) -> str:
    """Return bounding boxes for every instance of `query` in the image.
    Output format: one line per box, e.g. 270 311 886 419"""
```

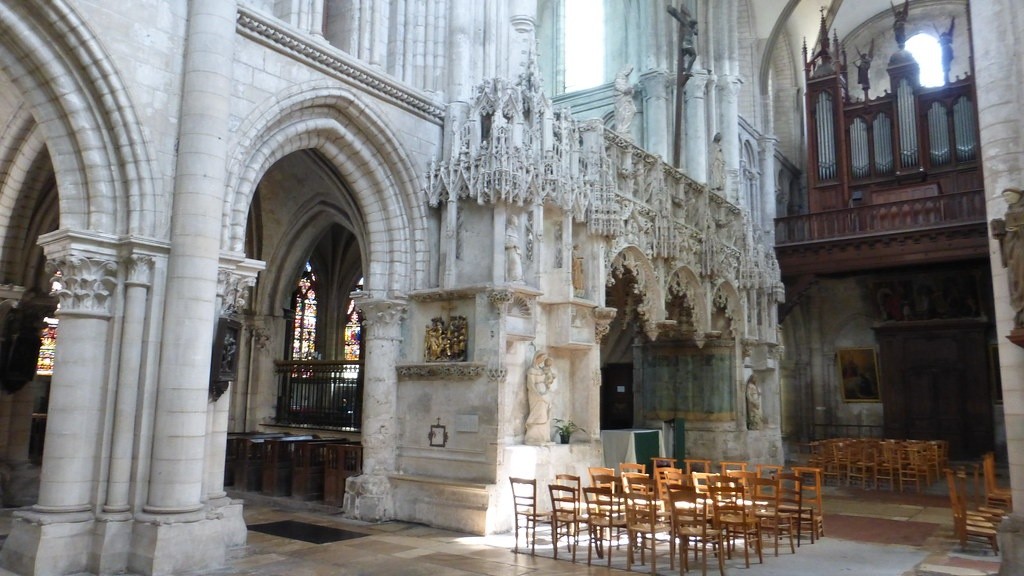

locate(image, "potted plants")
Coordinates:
550 417 587 444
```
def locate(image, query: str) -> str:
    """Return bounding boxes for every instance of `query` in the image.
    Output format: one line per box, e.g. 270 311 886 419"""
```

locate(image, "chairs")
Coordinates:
508 439 1012 576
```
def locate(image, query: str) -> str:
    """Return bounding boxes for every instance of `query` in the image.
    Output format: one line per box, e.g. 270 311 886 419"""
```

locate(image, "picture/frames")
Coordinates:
837 348 881 401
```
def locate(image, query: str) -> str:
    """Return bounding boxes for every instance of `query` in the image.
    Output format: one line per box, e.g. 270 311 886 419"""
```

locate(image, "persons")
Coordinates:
572 242 585 289
893 11 905 44
708 133 726 190
1002 186 1024 330
525 350 557 445
506 215 524 280
221 338 236 373
613 65 636 143
858 53 870 84
940 32 954 70
745 373 762 429
